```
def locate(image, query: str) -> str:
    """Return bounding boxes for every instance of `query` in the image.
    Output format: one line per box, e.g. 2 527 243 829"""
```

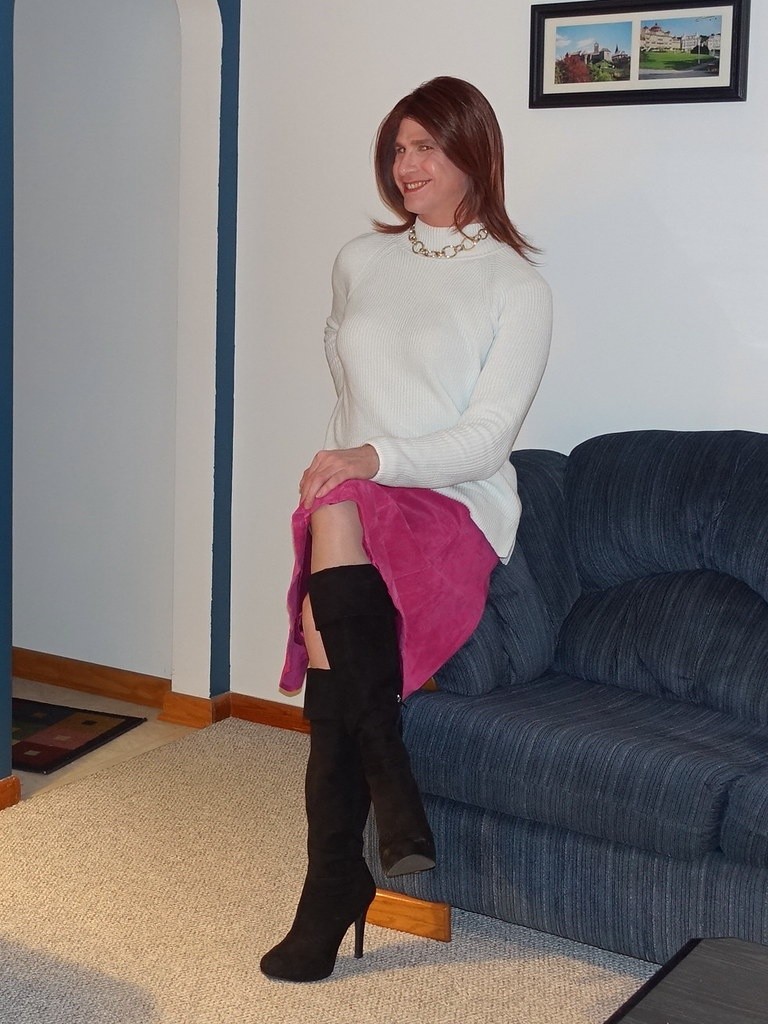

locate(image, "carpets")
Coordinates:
11 697 148 775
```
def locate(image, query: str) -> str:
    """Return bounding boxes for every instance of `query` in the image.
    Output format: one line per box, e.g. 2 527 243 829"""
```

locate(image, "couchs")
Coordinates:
364 429 768 966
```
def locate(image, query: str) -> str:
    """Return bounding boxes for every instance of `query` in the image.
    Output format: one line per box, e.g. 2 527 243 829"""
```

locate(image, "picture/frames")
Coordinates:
528 0 752 110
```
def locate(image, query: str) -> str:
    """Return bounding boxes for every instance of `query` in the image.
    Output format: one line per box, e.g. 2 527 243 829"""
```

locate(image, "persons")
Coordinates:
259 76 552 984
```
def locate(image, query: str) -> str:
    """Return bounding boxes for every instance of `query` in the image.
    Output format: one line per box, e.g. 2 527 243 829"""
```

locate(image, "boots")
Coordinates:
260 667 376 983
307 564 437 878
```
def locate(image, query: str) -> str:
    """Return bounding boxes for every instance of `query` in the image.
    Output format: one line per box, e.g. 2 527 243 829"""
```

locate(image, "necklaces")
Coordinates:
408 225 487 260
